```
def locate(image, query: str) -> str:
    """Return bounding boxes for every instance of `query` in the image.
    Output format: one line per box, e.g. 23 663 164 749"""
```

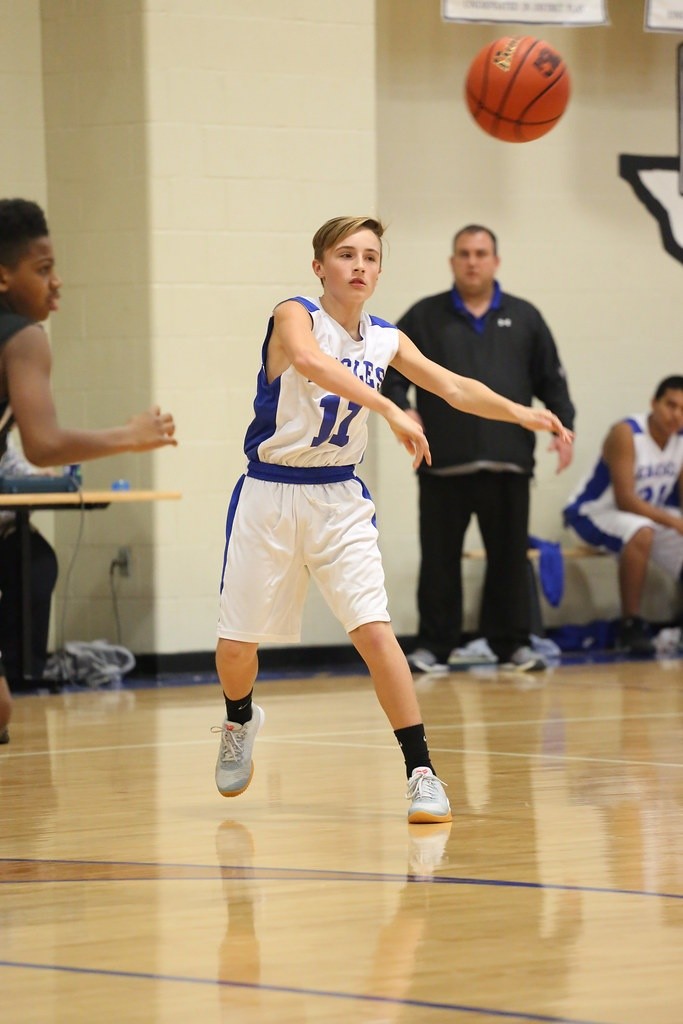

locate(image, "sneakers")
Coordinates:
405 767 453 825
618 615 660 660
216 704 263 798
406 647 451 672
502 646 547 671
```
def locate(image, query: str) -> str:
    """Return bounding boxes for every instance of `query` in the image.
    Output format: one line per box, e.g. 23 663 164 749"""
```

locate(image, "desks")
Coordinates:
0 480 180 688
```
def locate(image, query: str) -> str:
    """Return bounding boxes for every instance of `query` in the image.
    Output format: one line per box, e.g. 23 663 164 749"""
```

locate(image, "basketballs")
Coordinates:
465 34 568 143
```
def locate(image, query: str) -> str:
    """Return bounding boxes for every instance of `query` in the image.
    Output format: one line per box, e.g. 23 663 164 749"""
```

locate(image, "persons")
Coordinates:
0 419 65 694
213 216 576 828
1 196 180 743
378 222 577 676
558 375 683 657
